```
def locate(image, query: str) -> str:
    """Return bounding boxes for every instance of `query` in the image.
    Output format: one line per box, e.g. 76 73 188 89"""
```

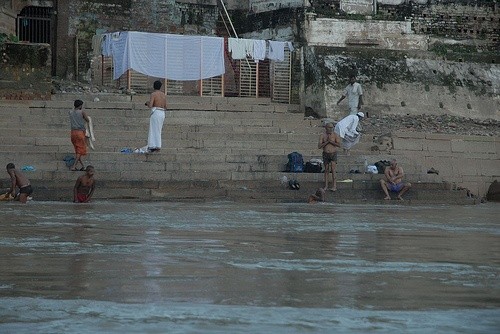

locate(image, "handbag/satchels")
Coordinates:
305 162 322 173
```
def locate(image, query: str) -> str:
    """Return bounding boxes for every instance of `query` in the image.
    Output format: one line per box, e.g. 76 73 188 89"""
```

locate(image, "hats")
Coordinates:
358 112 364 117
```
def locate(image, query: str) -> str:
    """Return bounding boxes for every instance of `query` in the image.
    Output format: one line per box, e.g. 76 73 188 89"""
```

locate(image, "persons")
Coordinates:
311 188 326 201
74 165 96 203
5 163 33 197
318 123 340 192
18 193 28 202
337 74 364 115
380 158 411 199
147 81 168 152
69 99 89 171
334 112 364 156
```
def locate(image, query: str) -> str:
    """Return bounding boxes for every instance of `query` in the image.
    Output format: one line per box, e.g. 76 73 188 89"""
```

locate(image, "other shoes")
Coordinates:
289 180 296 190
350 170 355 173
294 180 300 190
355 170 361 174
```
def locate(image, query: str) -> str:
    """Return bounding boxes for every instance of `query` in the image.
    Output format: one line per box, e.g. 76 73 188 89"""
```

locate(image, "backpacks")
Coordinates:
487 180 500 201
287 152 304 172
